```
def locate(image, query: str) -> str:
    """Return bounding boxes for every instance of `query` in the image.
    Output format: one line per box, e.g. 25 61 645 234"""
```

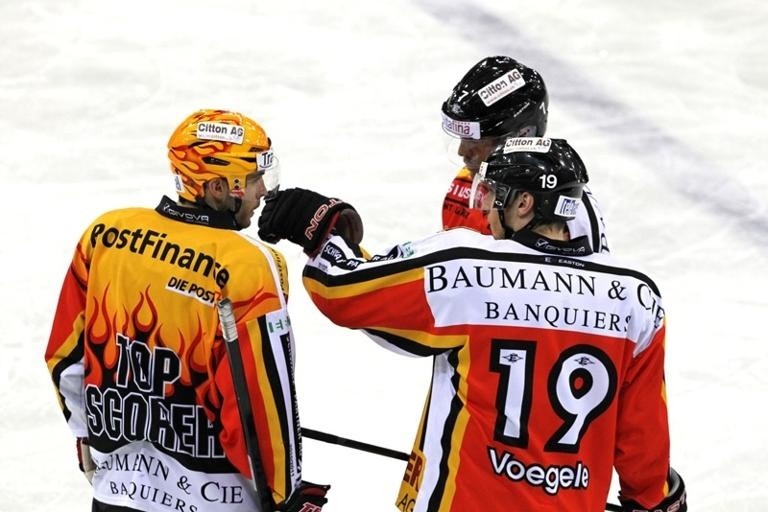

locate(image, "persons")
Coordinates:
258 136 688 511
441 55 610 254
44 108 331 512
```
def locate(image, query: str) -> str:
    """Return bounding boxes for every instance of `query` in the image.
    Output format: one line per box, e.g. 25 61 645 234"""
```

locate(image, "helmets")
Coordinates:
438 55 549 144
468 136 590 222
165 106 281 205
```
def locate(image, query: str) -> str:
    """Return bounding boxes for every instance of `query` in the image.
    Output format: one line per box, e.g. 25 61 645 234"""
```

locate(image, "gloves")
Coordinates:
75 437 97 485
617 466 689 512
262 479 331 512
257 187 367 259
